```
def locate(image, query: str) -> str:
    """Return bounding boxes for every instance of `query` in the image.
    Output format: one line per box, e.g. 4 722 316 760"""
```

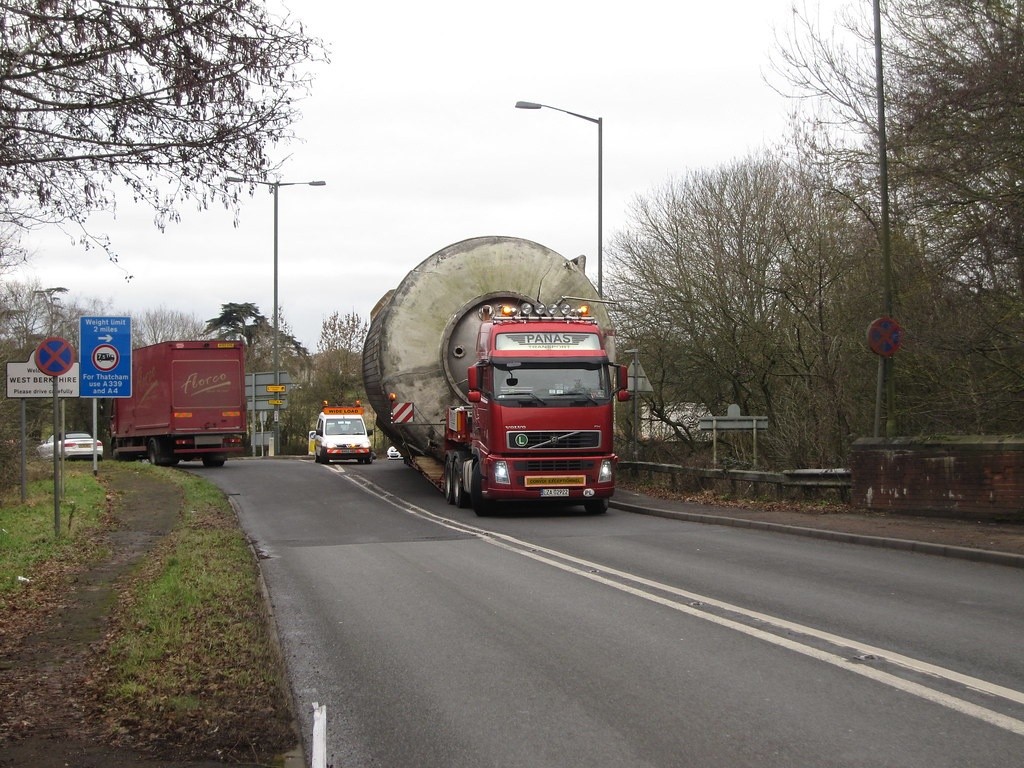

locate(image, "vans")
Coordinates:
314 415 372 463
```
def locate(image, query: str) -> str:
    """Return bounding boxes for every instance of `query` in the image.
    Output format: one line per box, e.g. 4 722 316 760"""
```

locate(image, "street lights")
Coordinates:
516 100 602 297
227 176 326 455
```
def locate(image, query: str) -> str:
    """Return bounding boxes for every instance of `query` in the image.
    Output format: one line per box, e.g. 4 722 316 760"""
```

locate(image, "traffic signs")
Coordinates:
79 316 133 398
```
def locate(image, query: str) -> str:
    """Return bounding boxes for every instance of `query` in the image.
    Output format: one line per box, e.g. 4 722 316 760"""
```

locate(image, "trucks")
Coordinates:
99 337 247 466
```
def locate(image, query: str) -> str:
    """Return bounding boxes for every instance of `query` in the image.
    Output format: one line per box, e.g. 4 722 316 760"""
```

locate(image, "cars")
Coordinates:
387 445 402 459
36 431 103 462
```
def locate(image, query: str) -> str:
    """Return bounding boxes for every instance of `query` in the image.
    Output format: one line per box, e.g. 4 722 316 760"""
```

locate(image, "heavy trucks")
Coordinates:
362 235 629 517
